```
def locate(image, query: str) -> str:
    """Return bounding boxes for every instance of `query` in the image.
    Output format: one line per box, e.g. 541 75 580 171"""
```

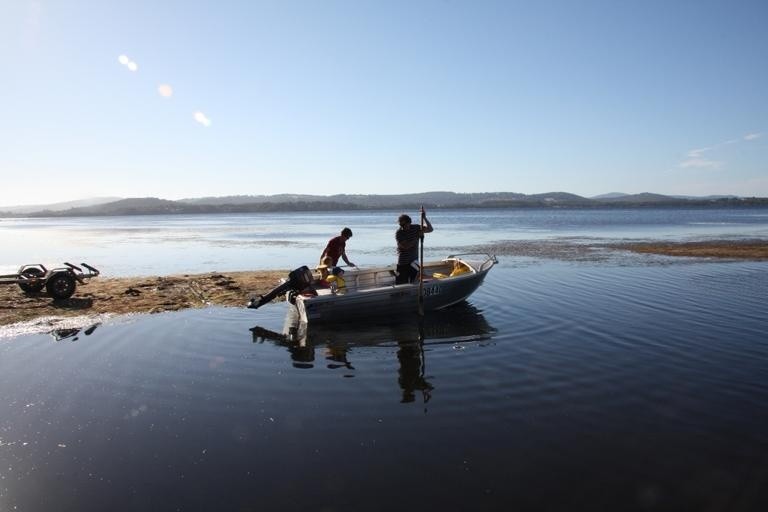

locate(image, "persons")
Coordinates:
317 256 333 288
327 267 345 288
396 342 434 402
320 227 356 267
395 208 433 285
325 342 354 372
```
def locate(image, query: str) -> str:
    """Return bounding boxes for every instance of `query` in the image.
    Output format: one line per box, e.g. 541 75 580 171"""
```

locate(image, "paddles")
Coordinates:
418 206 424 316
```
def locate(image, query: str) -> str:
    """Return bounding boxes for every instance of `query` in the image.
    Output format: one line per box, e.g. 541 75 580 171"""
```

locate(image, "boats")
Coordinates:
245 250 499 326
280 299 498 355
